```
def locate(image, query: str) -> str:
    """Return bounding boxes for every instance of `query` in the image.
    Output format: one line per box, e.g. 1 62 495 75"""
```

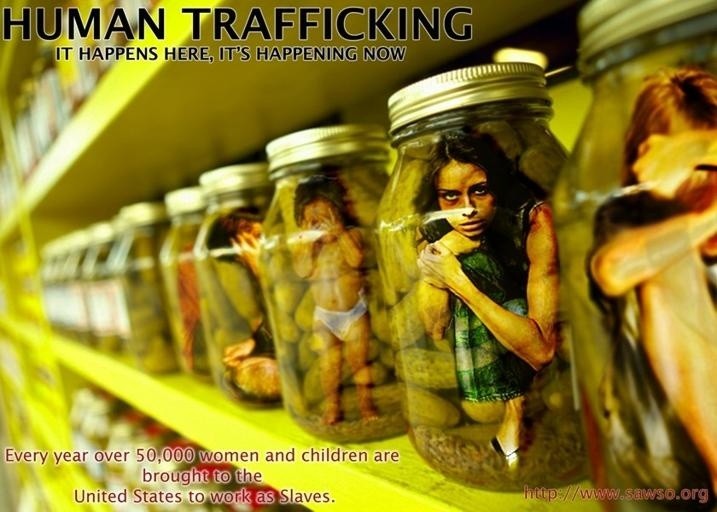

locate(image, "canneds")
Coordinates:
34 1 717 512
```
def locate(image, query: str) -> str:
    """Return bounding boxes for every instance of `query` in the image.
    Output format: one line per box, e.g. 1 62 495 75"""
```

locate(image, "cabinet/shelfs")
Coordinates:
0 0 717 512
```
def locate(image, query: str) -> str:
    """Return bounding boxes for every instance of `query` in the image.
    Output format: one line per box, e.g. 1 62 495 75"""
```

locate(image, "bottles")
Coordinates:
40 0 717 512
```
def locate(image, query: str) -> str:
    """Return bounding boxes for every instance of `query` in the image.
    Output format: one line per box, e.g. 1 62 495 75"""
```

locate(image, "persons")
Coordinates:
208 204 287 405
292 161 386 424
582 65 716 512
411 126 572 460
